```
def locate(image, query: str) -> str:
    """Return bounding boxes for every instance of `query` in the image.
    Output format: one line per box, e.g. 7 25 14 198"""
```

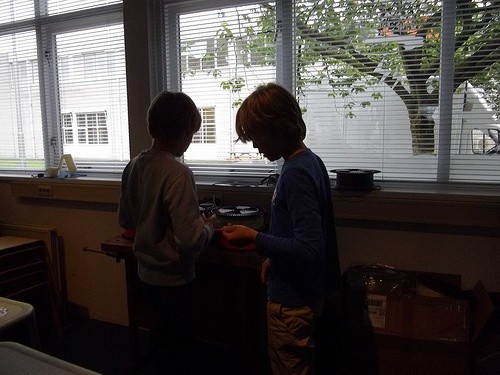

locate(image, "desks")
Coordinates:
100 234 267 375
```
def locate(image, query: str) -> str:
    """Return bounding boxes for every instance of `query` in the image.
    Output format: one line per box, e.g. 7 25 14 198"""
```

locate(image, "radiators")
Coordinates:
0 223 63 305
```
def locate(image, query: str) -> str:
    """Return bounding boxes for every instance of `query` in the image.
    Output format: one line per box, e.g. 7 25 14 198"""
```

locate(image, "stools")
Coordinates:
1 236 65 339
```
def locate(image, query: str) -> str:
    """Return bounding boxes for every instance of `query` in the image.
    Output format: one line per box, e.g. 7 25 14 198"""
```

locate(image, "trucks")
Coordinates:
227 60 500 186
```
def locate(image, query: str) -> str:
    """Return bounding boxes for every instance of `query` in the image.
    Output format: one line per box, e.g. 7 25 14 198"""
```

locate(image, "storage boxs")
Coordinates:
338 263 494 375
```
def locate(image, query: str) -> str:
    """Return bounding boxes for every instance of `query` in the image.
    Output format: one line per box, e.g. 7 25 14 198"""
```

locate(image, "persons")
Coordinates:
222 83 340 375
115 90 220 374
